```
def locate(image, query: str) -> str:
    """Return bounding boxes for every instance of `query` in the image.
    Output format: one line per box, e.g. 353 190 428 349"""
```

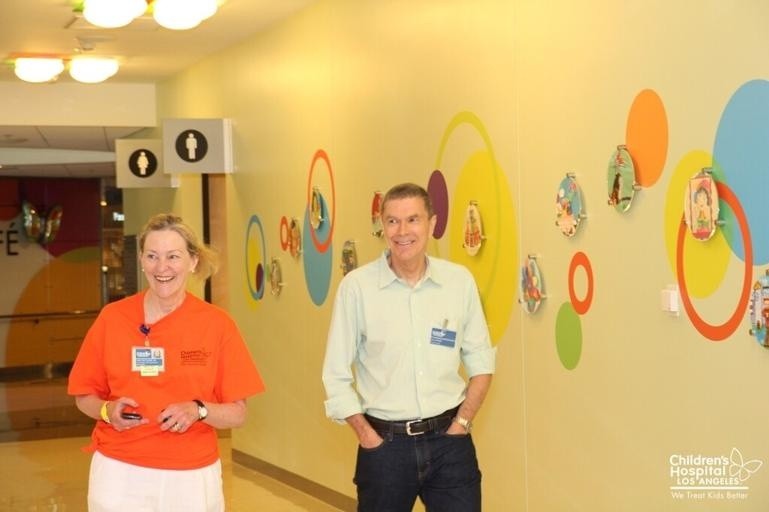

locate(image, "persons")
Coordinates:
65 213 267 512
322 182 496 511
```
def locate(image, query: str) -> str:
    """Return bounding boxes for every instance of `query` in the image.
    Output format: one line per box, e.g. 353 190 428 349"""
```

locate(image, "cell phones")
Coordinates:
122 413 142 420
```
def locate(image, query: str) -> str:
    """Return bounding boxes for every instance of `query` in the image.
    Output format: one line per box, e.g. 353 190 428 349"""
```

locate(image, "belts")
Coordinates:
364 408 458 436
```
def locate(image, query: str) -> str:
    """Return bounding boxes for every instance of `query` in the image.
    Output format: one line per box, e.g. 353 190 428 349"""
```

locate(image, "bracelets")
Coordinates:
100 399 111 425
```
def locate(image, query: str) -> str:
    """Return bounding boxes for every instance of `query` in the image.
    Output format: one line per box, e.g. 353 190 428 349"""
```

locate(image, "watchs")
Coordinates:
192 399 207 424
453 416 472 433
